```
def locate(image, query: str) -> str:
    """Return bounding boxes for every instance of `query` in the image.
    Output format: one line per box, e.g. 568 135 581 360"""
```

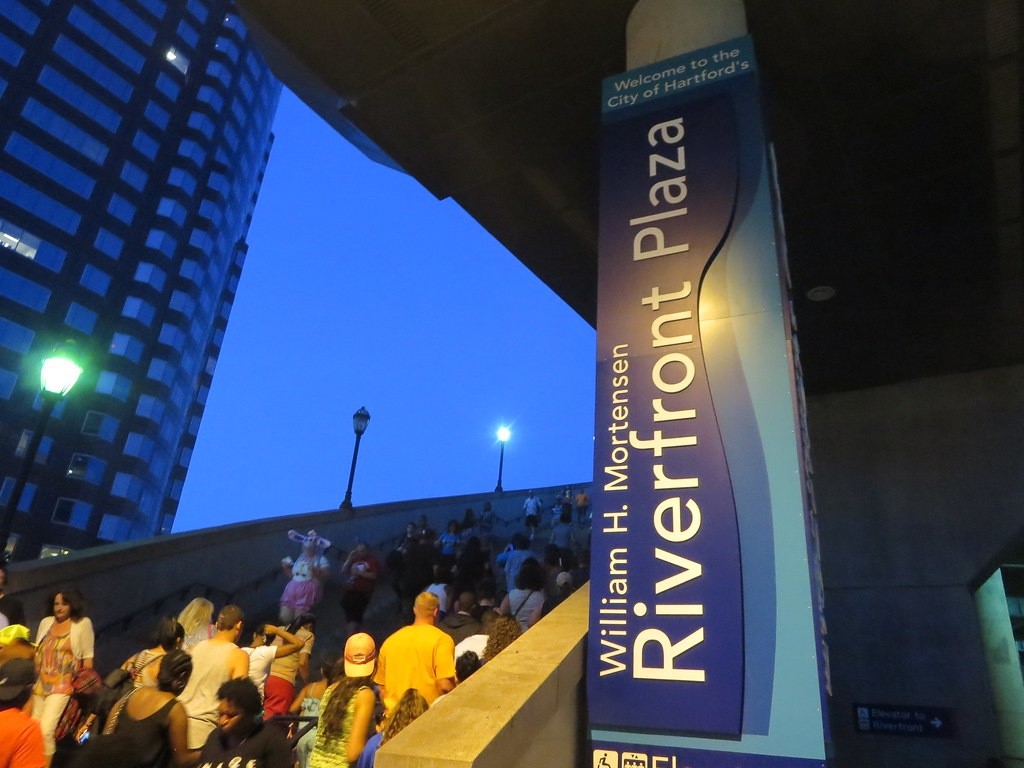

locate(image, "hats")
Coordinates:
344 633 378 677
1 658 37 701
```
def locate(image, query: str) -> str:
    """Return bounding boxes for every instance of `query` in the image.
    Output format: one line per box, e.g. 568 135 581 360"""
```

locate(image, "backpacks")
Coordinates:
97 649 163 711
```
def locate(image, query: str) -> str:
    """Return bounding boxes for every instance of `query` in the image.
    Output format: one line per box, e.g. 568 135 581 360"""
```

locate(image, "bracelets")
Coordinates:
85 722 93 727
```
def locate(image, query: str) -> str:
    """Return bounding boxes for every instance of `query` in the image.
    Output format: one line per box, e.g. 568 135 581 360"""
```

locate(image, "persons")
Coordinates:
0 484 592 768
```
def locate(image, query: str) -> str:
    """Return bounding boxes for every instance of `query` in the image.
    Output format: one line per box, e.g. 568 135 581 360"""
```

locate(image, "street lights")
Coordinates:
340 406 371 510
0 336 86 564
494 427 509 492
